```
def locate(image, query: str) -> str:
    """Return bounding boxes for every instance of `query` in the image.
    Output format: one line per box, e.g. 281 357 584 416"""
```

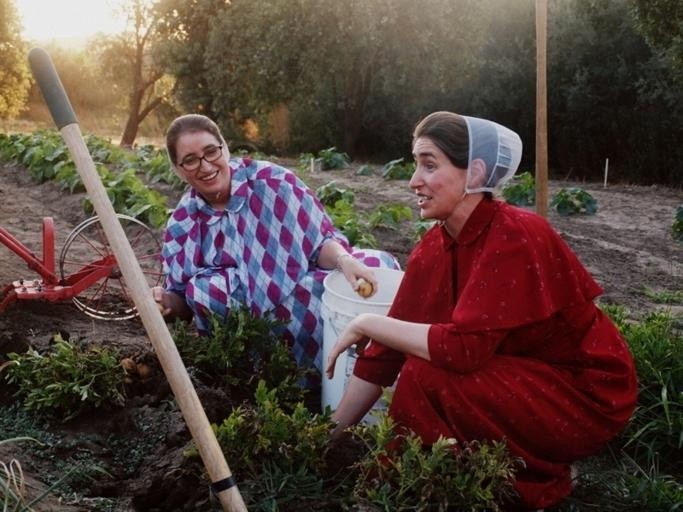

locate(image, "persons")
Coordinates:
308 109 639 504
134 113 407 378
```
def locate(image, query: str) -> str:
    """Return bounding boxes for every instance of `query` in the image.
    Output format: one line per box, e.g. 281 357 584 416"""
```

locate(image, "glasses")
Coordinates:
173 142 224 173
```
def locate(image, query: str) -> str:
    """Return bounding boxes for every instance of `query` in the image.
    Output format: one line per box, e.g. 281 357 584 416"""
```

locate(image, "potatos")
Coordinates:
356 278 372 298
120 358 151 387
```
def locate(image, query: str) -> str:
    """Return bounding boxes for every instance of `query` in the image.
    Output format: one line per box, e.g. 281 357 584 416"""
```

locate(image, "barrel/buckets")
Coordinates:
320 269 405 424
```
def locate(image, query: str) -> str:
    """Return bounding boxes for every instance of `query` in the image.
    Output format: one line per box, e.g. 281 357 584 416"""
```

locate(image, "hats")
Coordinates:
461 115 525 199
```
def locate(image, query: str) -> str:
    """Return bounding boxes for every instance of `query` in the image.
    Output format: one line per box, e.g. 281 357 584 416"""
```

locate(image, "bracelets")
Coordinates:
334 252 351 266
